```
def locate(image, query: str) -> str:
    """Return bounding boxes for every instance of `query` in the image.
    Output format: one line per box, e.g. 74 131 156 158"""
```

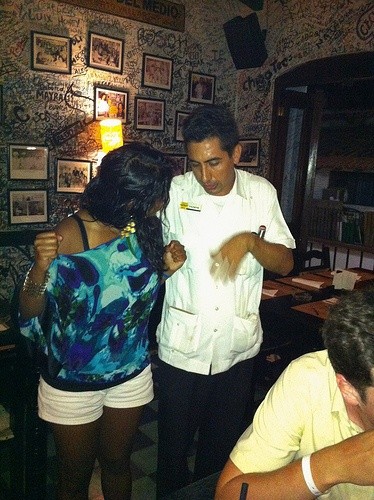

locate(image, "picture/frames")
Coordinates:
31 30 72 74
7 142 50 182
7 188 50 226
141 52 173 91
93 85 128 125
133 96 165 131
87 30 123 75
54 157 93 195
235 137 263 168
173 110 192 143
188 70 217 105
163 153 190 174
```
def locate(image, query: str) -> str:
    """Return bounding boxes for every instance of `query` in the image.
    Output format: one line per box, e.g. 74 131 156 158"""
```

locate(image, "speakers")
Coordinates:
222 13 267 70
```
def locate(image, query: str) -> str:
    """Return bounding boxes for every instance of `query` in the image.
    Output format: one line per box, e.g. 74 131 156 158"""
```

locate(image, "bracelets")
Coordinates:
21 261 50 297
161 272 171 279
301 455 328 496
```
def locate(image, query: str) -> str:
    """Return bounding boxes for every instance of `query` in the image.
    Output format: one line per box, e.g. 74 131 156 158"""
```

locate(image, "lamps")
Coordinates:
99 118 123 152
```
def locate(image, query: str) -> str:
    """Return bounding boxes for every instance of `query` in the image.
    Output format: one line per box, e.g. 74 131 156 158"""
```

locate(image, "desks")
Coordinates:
258 266 372 317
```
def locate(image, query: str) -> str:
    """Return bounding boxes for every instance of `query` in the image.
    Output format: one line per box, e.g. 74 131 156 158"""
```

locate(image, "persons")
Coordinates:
18 139 187 500
155 104 296 500
181 254 374 500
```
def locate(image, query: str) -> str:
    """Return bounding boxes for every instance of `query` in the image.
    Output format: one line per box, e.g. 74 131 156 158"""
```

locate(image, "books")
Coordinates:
308 207 374 248
